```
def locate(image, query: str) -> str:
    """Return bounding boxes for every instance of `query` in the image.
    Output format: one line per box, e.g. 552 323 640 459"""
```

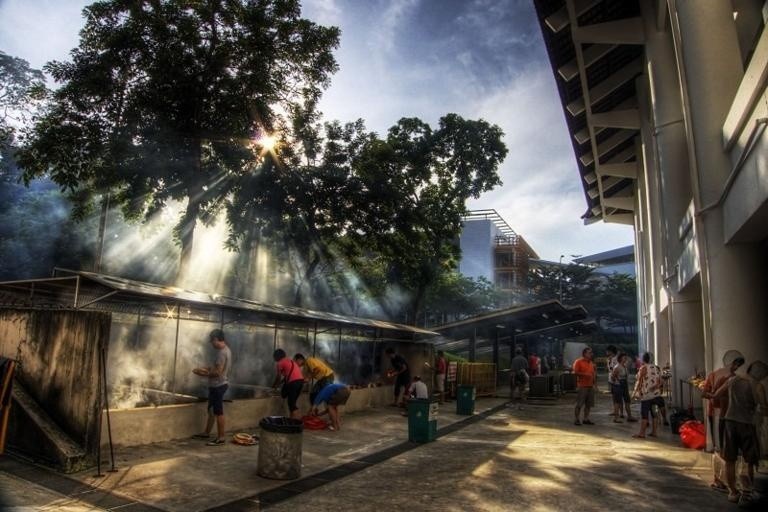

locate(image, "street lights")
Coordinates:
559 254 565 303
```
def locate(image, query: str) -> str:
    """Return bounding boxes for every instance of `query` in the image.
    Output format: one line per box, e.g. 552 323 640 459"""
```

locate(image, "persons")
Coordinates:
605 346 669 438
292 352 332 413
387 348 410 406
699 350 741 492
402 377 429 417
309 382 353 432
707 361 768 507
573 347 596 424
431 352 448 406
505 347 529 410
528 350 566 374
192 330 232 446
271 347 304 420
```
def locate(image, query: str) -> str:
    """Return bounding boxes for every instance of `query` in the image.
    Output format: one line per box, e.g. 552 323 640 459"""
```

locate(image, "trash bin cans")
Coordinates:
257 416 304 480
407 397 440 443
456 383 477 416
670 413 686 434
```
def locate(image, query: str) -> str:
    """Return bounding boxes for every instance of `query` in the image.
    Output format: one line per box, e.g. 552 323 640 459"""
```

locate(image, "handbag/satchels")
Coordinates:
670 412 696 434
192 367 210 376
259 416 304 433
410 390 416 399
281 384 288 399
520 368 529 385
233 433 259 445
679 419 705 448
303 415 328 430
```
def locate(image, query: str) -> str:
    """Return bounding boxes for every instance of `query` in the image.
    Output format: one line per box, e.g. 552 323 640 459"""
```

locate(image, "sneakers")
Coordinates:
608 413 638 423
575 419 581 425
207 437 224 446
192 433 209 440
505 402 525 410
583 419 594 424
632 420 668 438
711 475 759 506
